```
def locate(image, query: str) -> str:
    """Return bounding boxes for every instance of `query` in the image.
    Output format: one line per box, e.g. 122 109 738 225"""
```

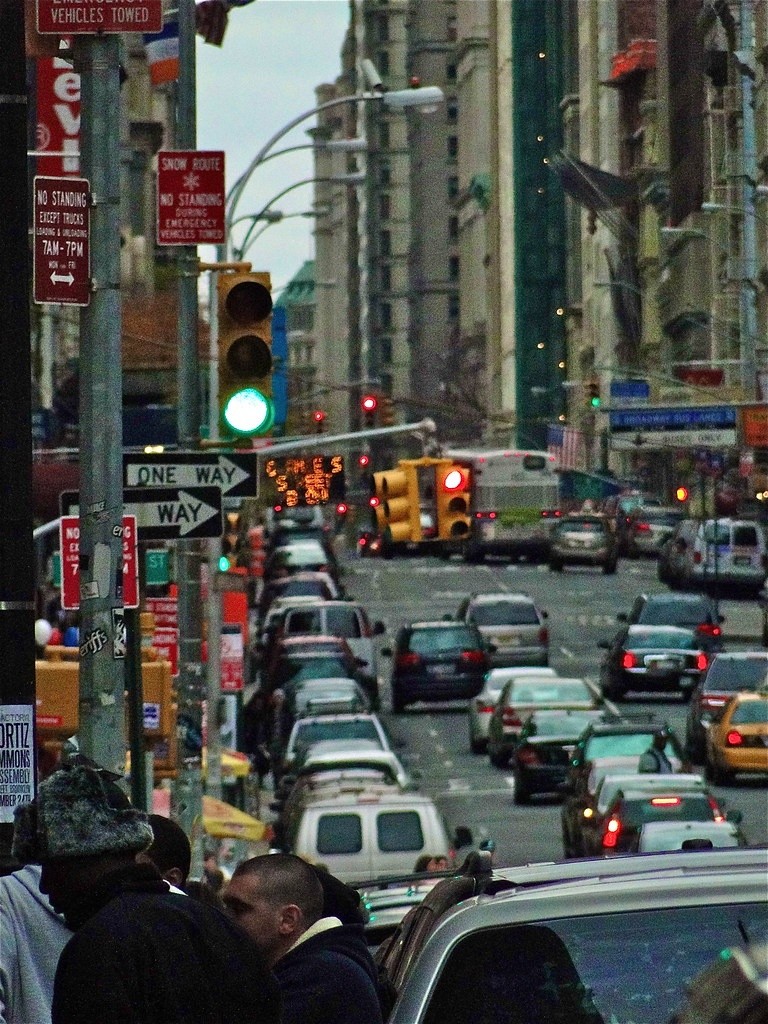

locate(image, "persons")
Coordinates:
416 825 498 871
14 758 271 1023
223 855 398 1024
637 729 674 774
0 862 191 1024
145 812 192 885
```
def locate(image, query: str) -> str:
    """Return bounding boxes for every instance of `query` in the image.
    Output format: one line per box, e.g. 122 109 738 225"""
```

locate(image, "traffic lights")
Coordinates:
301 410 329 435
582 378 601 410
364 396 378 429
380 398 396 425
219 509 242 574
370 461 420 544
217 273 274 444
437 461 472 542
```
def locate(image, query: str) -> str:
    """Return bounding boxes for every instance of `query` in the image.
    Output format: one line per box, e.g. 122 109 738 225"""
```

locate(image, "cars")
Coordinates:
617 589 725 654
700 687 768 785
597 625 707 704
549 511 619 576
379 621 501 715
254 489 746 932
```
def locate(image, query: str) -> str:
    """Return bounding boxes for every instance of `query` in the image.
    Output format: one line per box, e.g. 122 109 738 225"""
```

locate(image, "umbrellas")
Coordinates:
202 745 251 775
151 788 267 840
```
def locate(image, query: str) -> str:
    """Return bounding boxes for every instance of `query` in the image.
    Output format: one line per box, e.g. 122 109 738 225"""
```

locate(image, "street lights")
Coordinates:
207 84 448 866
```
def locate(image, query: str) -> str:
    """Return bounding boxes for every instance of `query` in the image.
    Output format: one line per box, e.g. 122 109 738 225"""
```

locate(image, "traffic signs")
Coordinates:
33 173 92 308
62 485 225 541
121 450 260 500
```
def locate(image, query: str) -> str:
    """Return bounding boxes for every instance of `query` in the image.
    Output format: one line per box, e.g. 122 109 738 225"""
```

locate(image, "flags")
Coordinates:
142 21 180 84
556 156 637 212
543 425 581 472
195 0 254 46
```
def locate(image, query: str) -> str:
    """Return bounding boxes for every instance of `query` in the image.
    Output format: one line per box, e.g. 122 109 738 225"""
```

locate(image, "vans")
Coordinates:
657 514 768 598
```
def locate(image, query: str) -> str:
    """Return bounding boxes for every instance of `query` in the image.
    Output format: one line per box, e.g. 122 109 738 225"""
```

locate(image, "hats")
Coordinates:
12 766 154 866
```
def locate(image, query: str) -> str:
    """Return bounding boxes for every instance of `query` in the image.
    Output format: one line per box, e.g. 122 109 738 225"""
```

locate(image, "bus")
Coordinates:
443 444 560 566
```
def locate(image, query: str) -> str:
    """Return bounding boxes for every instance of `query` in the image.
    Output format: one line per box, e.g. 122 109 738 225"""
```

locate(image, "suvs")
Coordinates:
441 590 551 671
375 846 768 1023
683 652 768 766
